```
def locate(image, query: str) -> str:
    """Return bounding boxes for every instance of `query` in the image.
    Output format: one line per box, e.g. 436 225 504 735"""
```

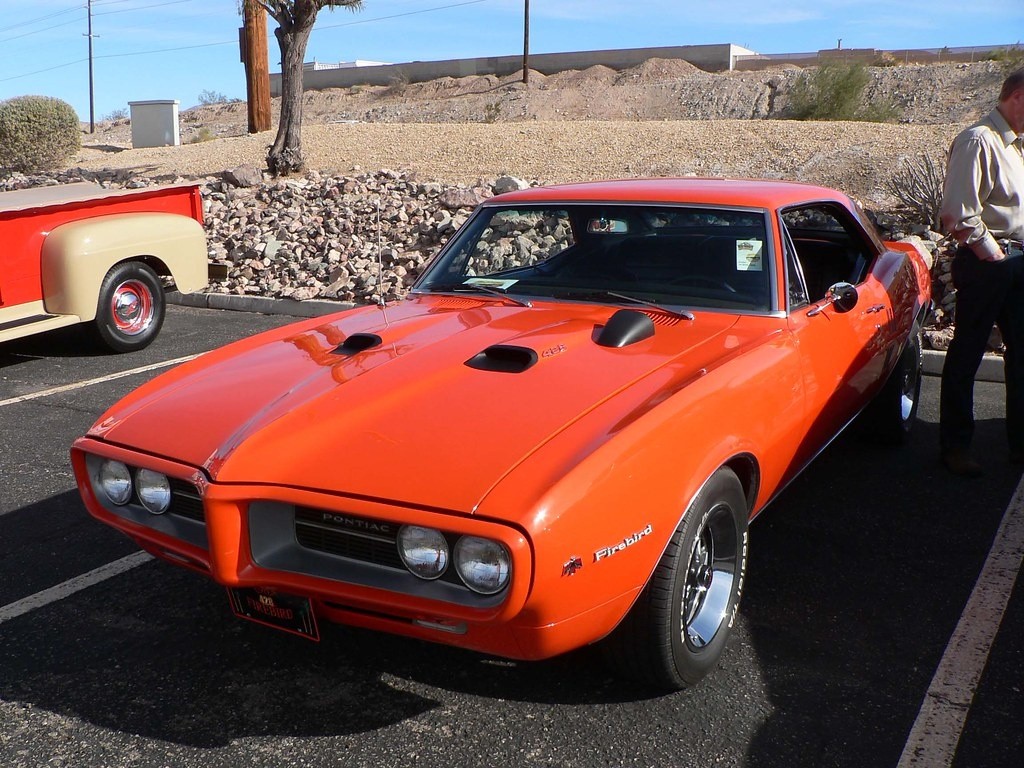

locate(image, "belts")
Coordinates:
957 242 1023 249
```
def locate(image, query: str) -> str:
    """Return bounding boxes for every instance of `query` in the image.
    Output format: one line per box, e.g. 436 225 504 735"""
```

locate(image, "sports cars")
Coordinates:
67 176 934 690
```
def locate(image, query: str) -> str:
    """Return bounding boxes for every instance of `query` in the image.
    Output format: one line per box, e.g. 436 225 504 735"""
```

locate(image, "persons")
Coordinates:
938 68 1024 476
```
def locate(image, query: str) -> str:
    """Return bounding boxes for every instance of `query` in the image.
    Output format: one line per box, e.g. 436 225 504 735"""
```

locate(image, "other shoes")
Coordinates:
941 450 982 477
1011 452 1024 464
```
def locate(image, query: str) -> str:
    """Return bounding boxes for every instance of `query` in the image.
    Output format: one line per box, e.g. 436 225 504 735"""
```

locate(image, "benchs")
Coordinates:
610 235 849 301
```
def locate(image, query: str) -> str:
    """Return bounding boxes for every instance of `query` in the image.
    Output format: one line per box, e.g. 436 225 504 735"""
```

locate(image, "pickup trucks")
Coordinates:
0 180 231 353
